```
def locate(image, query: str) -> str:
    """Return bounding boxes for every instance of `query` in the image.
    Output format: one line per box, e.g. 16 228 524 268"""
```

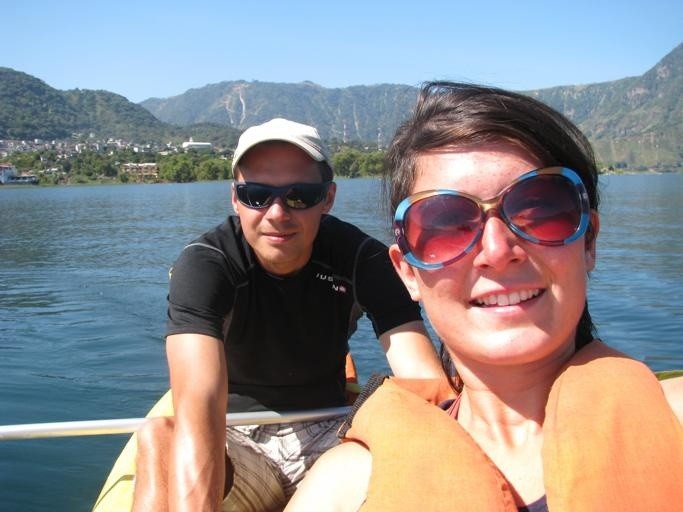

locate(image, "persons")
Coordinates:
134 117 452 511
280 80 683 511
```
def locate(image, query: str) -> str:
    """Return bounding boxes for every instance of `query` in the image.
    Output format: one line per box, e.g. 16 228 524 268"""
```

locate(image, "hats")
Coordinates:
231 118 328 178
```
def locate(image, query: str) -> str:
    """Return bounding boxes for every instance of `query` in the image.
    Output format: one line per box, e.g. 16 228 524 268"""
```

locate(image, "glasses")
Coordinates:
234 180 329 209
394 167 593 270
